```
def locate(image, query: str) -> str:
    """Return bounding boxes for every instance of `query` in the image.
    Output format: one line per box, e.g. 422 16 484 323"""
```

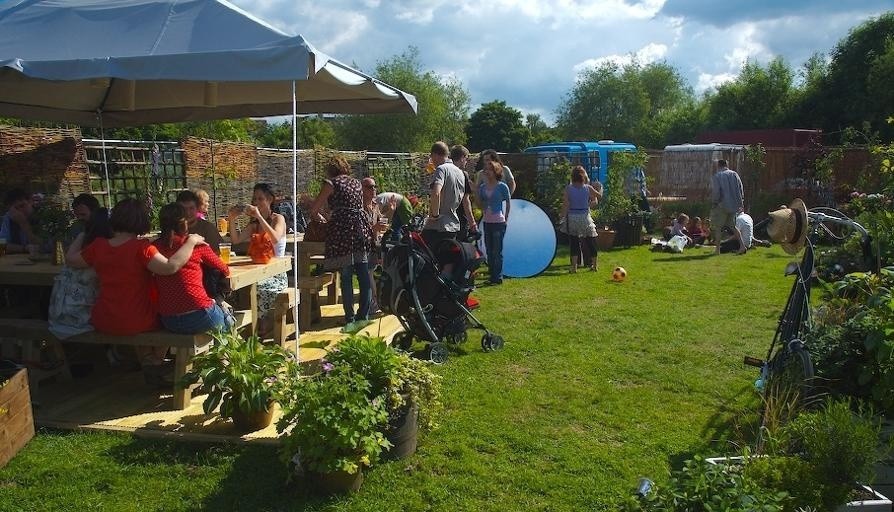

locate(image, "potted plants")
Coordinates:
183 320 290 431
269 368 387 496
587 189 635 251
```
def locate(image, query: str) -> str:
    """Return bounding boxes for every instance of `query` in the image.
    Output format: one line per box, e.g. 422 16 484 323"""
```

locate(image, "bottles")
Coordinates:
220 218 227 233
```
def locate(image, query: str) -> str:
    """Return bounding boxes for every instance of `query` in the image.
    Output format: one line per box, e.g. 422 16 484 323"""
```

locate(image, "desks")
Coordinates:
0 253 293 356
281 240 325 275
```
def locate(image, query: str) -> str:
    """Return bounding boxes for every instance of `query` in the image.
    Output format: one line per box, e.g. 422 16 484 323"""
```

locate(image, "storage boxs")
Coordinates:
0 357 37 471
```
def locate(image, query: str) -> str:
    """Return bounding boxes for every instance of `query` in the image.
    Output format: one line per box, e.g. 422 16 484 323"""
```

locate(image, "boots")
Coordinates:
589 255 598 272
567 256 577 275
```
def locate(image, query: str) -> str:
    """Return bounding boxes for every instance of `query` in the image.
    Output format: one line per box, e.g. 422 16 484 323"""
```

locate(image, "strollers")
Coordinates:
376 202 510 364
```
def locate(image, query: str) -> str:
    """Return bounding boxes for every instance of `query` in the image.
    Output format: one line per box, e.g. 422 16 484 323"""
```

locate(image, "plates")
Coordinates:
12 261 34 265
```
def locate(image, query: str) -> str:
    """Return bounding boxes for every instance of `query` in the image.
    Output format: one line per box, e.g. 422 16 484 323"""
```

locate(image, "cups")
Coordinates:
0 237 6 256
379 217 388 224
218 243 231 264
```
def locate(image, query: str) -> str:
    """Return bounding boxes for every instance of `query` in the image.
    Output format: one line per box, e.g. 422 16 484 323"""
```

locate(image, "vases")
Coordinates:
379 400 421 459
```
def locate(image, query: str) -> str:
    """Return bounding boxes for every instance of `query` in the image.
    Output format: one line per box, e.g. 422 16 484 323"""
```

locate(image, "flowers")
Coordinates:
318 332 438 405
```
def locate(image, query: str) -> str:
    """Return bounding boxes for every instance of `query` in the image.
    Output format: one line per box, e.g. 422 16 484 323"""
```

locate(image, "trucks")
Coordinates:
658 142 753 196
522 138 649 203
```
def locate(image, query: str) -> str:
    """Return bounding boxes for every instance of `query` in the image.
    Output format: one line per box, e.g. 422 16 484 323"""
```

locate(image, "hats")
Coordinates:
769 199 807 254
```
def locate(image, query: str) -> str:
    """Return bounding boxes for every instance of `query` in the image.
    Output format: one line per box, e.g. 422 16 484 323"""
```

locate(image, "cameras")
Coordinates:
236 204 246 212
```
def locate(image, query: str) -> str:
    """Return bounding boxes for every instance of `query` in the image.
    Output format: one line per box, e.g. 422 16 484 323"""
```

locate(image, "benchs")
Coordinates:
1 310 216 412
286 275 332 331
240 285 303 347
309 255 341 304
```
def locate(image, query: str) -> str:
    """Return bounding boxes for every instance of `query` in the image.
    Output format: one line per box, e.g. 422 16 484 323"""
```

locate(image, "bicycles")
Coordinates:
743 203 876 421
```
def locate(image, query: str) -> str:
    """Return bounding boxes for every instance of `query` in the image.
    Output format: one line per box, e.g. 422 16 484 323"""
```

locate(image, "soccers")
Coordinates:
612 267 627 281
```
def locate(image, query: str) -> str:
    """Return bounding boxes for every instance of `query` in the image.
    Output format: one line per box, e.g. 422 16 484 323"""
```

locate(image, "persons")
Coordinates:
577 175 598 267
668 160 754 256
560 165 604 274
